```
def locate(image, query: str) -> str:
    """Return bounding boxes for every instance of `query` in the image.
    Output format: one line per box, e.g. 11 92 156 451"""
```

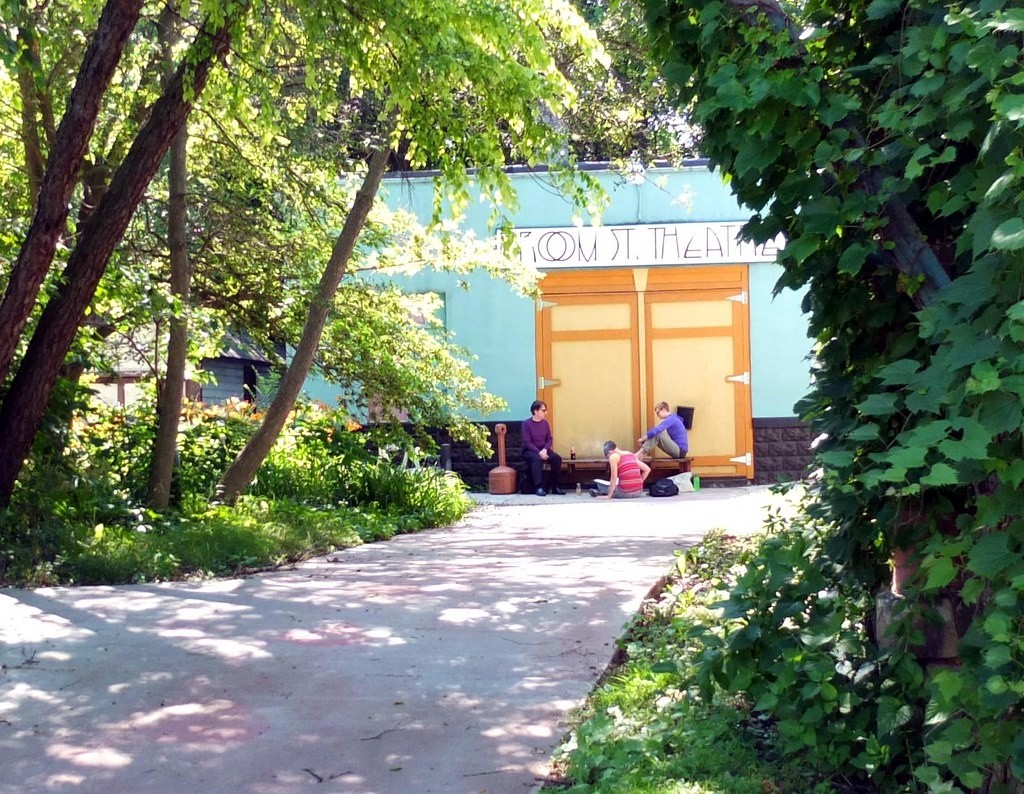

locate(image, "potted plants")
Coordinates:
891 512 934 599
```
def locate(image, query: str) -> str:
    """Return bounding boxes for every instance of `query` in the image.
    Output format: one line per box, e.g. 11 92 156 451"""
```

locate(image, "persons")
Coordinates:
521 400 566 496
590 441 651 499
635 402 688 460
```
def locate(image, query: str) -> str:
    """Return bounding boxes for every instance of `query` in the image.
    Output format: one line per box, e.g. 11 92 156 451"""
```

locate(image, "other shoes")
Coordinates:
552 488 566 494
537 488 546 495
589 489 599 497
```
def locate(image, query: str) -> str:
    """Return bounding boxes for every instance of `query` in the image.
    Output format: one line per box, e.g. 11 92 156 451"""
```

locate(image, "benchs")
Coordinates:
542 456 694 481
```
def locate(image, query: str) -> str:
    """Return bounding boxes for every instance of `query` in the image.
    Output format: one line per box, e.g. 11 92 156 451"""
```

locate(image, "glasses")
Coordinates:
539 409 547 413
656 410 660 415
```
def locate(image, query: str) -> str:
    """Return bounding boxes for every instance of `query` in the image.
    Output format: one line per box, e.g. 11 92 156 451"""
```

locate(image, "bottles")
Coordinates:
570 444 576 460
693 474 700 491
576 483 581 495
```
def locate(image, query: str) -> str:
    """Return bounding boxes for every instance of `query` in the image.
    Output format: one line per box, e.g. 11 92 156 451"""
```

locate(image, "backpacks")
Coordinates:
649 478 679 497
